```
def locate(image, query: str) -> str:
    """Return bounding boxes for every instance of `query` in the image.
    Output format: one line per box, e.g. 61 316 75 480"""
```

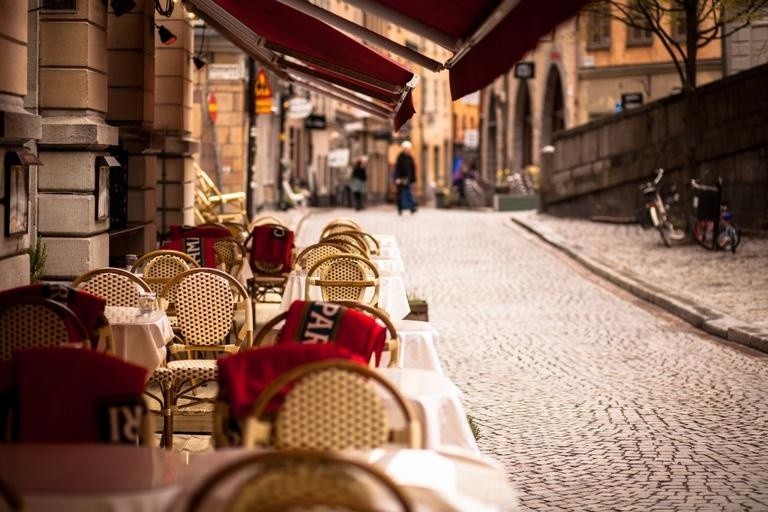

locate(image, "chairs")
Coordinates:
213 299 418 445
161 216 300 322
158 268 255 444
192 161 251 241
166 445 416 512
284 216 383 303
2 281 157 447
72 250 201 306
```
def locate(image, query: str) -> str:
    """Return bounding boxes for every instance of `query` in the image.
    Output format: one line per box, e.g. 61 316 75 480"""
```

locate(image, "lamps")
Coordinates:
150 17 176 46
186 51 204 70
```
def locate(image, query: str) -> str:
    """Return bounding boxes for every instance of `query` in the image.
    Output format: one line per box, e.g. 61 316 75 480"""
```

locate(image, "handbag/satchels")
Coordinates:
395 177 409 187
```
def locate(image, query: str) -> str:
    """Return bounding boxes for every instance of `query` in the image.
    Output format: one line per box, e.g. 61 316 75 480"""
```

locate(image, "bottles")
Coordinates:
291 248 306 271
139 293 156 314
126 254 136 274
615 100 621 112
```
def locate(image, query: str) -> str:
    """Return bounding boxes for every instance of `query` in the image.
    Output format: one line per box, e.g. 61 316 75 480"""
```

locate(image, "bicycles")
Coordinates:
687 167 741 255
638 168 687 248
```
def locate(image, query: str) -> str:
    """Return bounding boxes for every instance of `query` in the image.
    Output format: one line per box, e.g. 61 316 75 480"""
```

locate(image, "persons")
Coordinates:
351 158 367 212
452 151 470 207
394 140 417 216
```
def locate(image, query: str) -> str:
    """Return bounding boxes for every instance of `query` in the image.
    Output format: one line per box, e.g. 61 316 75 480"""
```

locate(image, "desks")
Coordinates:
1 447 522 512
